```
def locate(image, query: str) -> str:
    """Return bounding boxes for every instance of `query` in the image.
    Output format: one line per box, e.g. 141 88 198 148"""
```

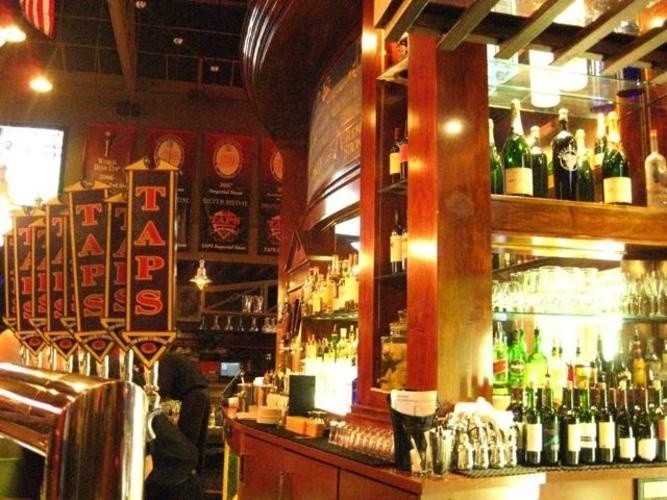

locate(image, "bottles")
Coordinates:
492 321 667 467
389 120 408 186
390 209 407 273
488 98 667 208
304 324 358 369
302 253 359 316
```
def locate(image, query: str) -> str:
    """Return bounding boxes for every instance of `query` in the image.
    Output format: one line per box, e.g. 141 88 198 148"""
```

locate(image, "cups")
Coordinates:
242 294 264 313
328 420 394 461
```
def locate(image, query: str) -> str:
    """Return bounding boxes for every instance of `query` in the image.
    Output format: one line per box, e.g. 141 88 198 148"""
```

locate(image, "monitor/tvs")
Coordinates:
219 360 244 379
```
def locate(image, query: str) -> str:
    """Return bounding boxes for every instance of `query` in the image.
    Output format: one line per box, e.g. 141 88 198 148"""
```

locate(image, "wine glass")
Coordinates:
198 313 277 333
491 266 667 317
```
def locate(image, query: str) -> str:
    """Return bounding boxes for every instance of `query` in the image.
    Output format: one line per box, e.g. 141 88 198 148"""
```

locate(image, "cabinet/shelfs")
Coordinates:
232 421 422 500
355 0 667 412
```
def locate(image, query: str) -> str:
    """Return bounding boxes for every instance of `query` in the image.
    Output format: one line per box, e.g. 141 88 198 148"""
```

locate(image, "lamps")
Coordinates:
189 258 213 290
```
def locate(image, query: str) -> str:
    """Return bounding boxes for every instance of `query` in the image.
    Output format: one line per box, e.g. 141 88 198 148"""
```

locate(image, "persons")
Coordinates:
135 349 211 453
